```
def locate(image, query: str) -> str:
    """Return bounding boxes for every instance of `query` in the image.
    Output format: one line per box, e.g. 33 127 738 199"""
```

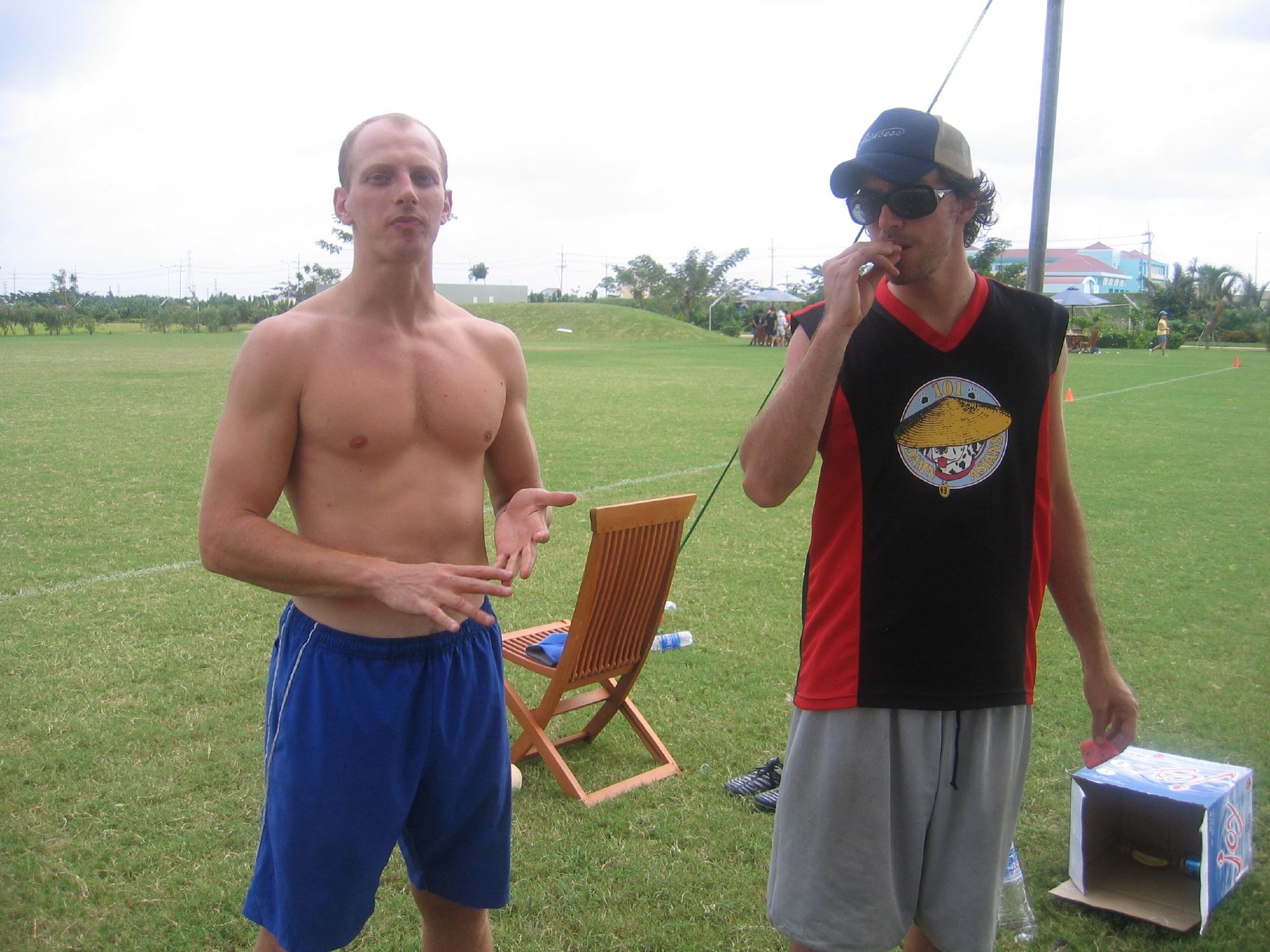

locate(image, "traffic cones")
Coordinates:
1233 357 1240 367
1065 388 1074 402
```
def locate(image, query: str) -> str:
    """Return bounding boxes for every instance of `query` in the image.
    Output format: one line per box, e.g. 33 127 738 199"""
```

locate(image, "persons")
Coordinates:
748 307 791 347
196 113 579 952
739 107 1139 950
1148 310 1170 357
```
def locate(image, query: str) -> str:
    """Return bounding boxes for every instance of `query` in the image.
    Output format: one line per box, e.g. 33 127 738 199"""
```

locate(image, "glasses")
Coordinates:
847 184 952 225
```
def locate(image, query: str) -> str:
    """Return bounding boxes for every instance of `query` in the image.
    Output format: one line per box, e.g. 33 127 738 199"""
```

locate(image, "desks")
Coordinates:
1065 335 1085 353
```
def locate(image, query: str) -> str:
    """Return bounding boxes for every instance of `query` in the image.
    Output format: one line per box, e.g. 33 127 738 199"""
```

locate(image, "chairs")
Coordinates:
501 492 699 807
1069 328 1099 352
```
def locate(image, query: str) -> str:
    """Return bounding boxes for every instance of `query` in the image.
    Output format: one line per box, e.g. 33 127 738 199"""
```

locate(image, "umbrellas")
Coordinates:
740 286 807 307
1049 286 1112 348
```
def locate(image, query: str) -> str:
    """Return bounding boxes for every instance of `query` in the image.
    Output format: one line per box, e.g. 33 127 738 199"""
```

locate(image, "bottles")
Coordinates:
650 631 693 651
664 600 676 613
998 841 1038 946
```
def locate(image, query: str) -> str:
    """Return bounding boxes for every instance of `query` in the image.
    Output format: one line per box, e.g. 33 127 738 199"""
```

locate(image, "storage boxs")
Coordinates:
1048 744 1255 936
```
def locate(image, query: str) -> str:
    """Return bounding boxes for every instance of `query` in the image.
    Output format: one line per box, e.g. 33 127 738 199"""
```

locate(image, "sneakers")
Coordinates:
724 758 783 794
1149 349 1153 355
751 787 778 809
1162 354 1169 357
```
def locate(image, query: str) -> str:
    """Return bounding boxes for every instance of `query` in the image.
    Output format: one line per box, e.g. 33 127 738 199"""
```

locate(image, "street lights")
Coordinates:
708 285 737 332
0 266 16 294
159 265 170 297
282 260 297 282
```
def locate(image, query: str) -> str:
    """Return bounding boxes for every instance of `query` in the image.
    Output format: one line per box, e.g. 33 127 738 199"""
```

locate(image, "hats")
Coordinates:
1160 311 1167 315
777 310 785 316
829 107 974 199
